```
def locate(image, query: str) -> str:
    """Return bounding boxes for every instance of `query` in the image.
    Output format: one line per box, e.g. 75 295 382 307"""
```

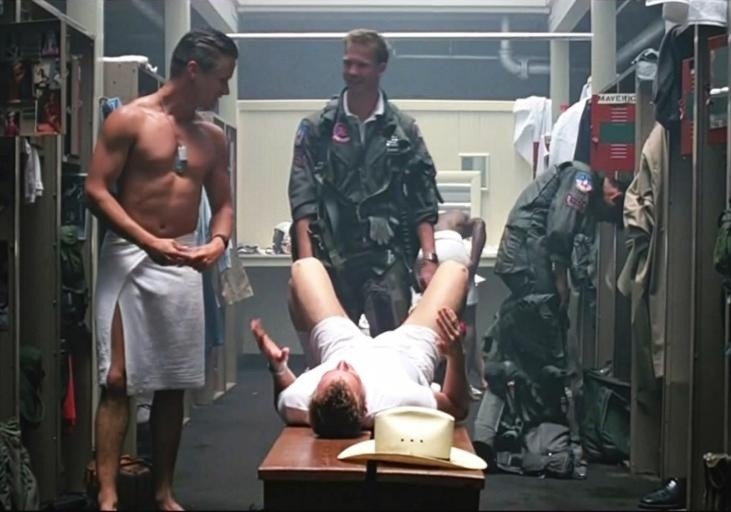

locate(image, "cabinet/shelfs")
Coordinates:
0 0 238 512
434 170 480 217
569 26 731 511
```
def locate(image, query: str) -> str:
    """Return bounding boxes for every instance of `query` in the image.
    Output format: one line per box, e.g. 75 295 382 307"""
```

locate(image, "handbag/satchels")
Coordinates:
520 421 575 476
580 359 631 463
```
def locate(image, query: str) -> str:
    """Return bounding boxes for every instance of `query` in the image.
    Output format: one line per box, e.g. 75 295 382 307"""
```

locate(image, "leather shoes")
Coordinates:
639 479 687 510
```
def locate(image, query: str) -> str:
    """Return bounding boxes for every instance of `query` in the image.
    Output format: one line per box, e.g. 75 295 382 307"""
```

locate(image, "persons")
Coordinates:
493 163 629 430
249 257 469 439
285 27 444 339
413 208 489 400
83 28 234 512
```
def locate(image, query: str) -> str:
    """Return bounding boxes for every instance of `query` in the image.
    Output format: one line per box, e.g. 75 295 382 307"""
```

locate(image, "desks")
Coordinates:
239 250 304 359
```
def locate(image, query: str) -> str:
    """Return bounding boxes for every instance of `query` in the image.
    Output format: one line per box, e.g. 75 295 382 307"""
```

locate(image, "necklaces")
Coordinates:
158 87 193 173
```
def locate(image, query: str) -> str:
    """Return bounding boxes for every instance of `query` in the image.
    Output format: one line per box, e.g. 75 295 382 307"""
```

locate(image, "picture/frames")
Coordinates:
458 153 490 192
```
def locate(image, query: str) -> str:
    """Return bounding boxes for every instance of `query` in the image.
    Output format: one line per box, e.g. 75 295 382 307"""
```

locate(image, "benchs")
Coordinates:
259 423 485 512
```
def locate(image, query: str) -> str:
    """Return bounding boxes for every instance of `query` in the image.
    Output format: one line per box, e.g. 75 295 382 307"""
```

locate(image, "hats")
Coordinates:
338 404 488 471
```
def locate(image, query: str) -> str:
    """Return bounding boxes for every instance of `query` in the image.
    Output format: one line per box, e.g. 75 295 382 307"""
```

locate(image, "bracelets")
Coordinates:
265 362 288 376
424 253 441 264
209 234 229 250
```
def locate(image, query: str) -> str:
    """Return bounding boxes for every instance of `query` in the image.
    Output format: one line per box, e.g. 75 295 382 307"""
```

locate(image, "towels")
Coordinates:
510 94 551 178
